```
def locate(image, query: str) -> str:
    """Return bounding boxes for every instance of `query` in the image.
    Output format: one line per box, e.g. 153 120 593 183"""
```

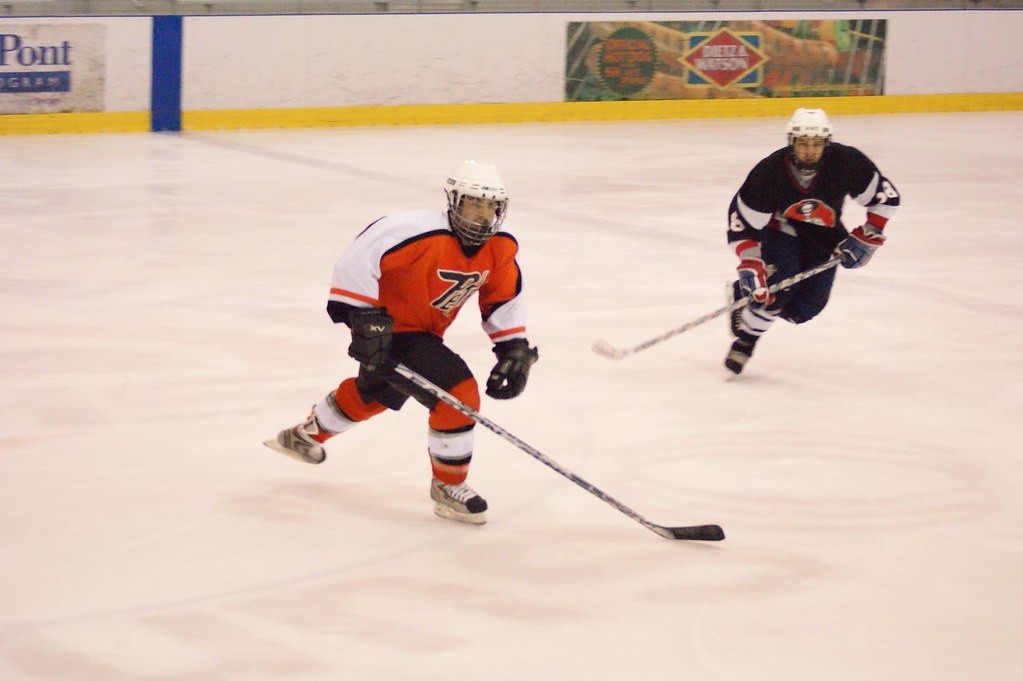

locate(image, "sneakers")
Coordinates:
727 281 751 337
721 330 759 379
263 414 325 464
428 447 487 524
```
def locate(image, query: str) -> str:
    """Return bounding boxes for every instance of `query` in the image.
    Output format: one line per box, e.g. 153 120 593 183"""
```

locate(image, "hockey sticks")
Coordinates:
385 356 726 543
589 252 843 359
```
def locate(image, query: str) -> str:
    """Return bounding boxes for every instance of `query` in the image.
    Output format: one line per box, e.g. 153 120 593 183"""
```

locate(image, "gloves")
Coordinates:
833 227 886 268
348 307 394 372
486 340 538 400
737 257 777 310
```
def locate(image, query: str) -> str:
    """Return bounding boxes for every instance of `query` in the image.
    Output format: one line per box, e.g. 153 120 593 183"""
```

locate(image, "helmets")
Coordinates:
786 108 833 170
444 163 510 258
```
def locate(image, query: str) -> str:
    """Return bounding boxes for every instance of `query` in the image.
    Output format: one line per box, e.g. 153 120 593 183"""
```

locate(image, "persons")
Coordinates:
277 160 539 513
724 108 901 375
